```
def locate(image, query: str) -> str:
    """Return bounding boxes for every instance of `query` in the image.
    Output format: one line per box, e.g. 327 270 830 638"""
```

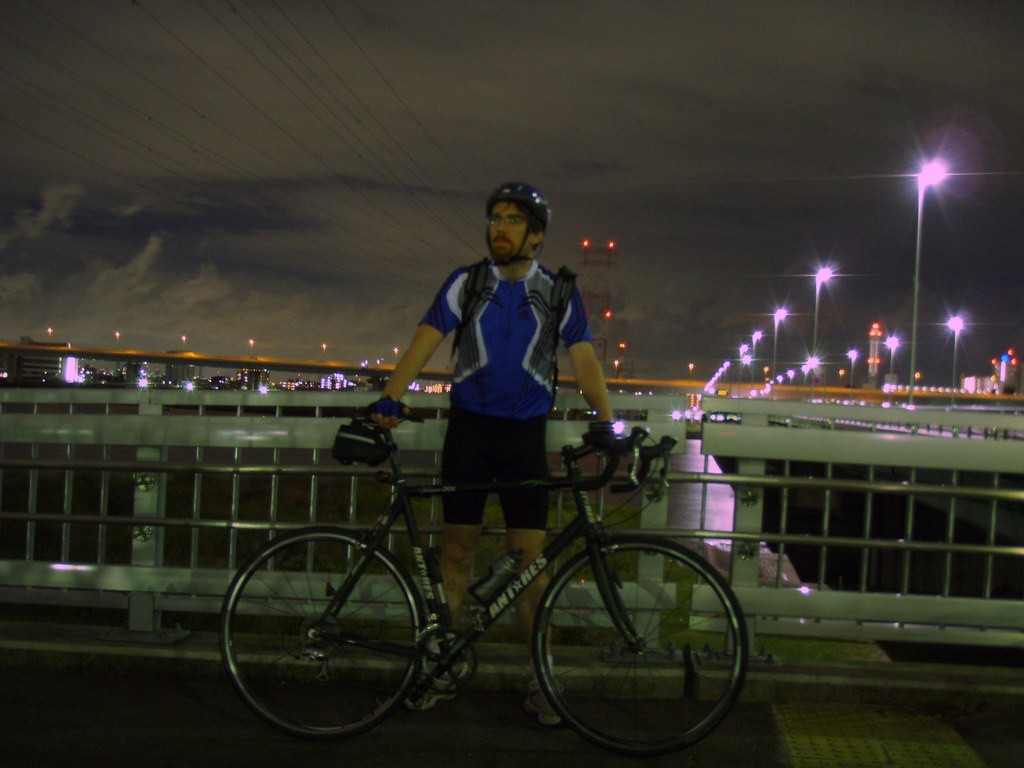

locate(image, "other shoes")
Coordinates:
525 681 566 724
405 689 458 713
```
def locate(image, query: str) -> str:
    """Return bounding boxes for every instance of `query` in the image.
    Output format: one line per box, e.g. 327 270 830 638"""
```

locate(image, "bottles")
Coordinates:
468 548 523 606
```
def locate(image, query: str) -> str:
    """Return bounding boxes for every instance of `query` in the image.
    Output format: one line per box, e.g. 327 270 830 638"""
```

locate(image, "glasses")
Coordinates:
486 213 530 225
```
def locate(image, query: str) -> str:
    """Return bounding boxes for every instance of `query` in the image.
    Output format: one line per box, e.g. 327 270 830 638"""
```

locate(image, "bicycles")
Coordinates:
215 401 752 759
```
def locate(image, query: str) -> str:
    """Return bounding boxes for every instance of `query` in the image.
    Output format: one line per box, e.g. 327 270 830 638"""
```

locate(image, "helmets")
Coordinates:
485 179 553 224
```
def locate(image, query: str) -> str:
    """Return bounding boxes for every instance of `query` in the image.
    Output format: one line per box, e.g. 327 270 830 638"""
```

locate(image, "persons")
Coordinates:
371 181 615 726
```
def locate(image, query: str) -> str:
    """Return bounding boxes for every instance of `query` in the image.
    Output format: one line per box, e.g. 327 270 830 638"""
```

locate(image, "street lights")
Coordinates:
809 266 833 401
904 163 948 408
847 350 858 388
769 307 788 399
884 336 900 408
946 316 966 413
735 343 747 400
748 330 763 398
